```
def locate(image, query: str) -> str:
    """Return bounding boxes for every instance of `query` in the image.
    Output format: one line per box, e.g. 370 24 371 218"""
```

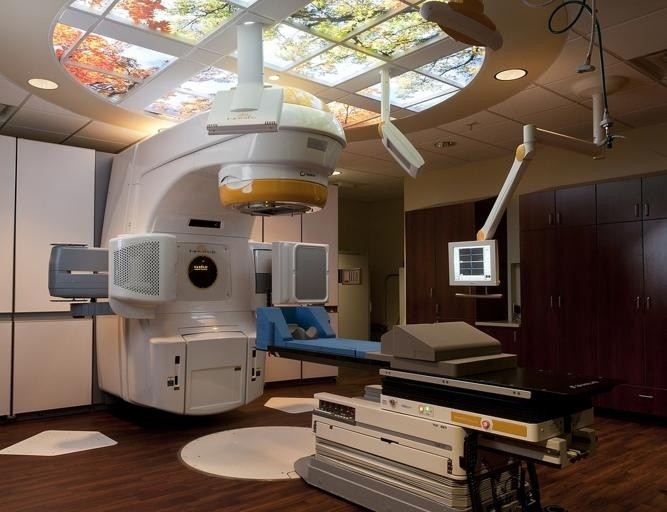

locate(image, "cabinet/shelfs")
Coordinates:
0 135 96 420
514 169 666 427
250 184 338 390
404 195 507 326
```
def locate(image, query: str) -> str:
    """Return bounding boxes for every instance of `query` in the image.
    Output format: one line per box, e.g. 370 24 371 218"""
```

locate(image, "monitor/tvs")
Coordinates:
448 240 499 287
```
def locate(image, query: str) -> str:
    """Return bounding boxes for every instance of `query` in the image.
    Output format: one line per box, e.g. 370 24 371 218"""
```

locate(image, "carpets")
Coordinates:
0 428 119 457
178 424 316 483
263 396 318 414
